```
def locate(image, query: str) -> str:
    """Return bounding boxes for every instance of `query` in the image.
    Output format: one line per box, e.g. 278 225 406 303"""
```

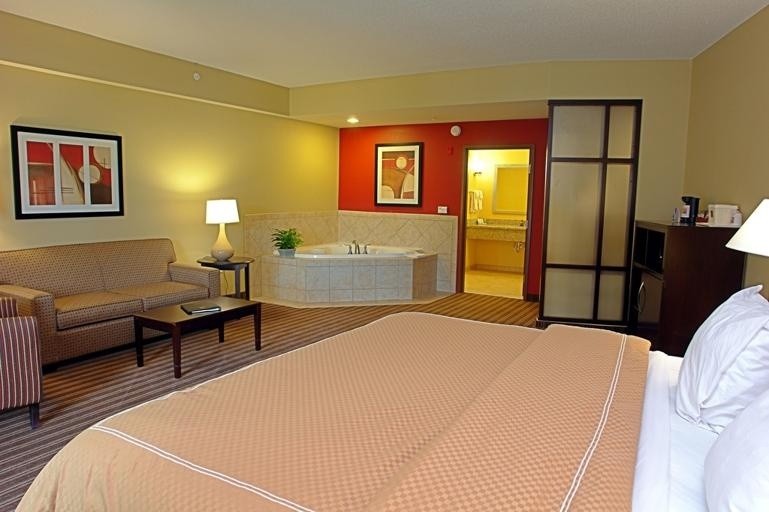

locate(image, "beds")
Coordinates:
14 284 769 512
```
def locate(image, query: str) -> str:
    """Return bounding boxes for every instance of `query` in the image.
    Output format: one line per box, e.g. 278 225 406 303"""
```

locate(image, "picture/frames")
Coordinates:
375 142 422 207
10 125 124 220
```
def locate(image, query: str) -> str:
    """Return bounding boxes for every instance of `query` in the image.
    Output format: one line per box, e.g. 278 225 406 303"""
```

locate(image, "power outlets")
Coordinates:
438 206 447 213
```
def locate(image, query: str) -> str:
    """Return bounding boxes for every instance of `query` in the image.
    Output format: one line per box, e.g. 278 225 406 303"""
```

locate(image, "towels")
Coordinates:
469 189 483 212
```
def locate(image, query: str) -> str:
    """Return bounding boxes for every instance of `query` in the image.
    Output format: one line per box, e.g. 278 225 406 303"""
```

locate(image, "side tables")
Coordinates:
196 256 254 320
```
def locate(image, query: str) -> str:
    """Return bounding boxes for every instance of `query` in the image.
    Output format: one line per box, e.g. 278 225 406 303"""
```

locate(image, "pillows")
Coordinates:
703 390 769 512
674 285 769 435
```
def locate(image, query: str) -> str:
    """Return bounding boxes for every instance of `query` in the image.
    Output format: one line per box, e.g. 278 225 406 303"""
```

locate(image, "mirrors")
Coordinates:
491 163 528 215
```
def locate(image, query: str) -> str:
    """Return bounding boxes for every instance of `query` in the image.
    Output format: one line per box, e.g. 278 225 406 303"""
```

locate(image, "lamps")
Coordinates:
205 199 241 261
725 198 769 258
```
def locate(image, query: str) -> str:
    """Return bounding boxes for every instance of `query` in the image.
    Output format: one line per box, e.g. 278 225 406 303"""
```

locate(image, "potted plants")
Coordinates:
270 227 304 257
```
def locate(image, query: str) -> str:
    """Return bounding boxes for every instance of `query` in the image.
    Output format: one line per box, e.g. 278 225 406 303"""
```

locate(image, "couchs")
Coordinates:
0 238 221 365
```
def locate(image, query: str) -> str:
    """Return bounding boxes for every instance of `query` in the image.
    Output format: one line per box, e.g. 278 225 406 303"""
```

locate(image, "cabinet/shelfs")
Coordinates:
628 220 745 357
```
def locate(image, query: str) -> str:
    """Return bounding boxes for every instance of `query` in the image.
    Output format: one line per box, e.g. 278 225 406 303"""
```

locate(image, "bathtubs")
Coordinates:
294 245 407 256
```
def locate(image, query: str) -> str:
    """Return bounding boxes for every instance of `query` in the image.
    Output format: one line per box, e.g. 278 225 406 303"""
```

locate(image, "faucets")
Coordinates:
352 239 360 254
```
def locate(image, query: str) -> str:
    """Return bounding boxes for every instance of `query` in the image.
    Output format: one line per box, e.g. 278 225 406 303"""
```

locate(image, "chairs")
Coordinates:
0 296 42 430
